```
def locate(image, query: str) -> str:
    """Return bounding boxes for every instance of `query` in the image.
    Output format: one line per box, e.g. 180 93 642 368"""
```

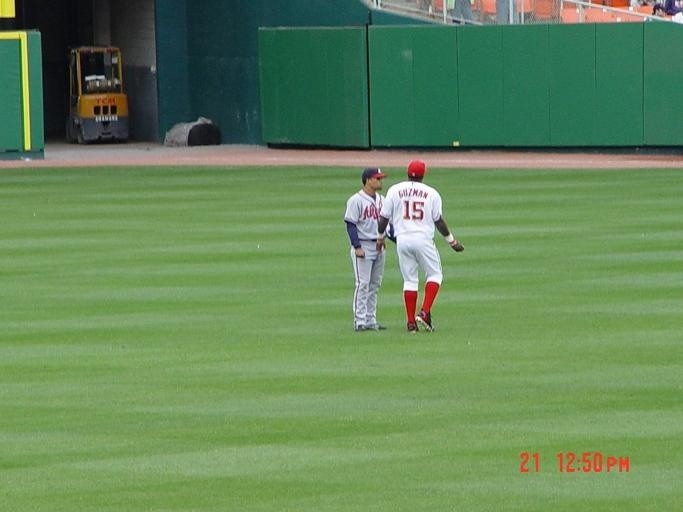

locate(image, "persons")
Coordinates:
628 0 683 23
376 160 464 331
495 1 519 24
446 0 474 25
344 167 396 331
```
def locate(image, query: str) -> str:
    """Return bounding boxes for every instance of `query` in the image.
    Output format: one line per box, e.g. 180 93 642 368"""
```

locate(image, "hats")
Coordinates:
409 161 425 176
363 168 385 178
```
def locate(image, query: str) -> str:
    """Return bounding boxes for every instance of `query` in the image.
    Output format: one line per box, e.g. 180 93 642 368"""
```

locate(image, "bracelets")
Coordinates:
376 231 385 239
444 232 454 242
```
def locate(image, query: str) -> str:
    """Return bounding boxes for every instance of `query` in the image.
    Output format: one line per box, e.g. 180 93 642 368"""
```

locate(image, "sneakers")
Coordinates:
407 322 418 333
356 326 369 330
367 324 382 330
416 309 432 331
